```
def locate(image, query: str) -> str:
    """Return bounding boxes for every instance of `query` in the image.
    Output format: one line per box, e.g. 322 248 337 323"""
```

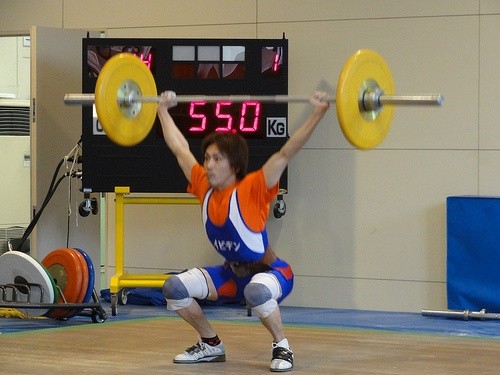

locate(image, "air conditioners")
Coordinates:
0 99 32 255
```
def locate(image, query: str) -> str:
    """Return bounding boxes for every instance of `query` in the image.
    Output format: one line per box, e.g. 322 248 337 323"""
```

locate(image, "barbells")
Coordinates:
64 48 442 151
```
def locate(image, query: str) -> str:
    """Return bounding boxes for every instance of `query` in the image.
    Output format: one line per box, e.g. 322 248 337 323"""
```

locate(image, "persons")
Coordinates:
156 91 330 371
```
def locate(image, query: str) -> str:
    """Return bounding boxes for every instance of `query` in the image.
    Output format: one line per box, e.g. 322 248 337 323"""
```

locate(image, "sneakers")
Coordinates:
173 339 226 363
270 342 295 372
